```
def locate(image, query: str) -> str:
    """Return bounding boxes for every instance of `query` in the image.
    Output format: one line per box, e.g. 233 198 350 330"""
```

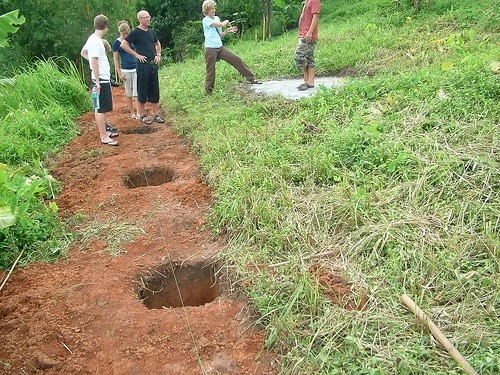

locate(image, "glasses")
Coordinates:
140 16 151 20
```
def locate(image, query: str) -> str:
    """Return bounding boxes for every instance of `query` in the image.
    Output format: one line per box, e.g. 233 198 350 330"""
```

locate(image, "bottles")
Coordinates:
91 84 101 110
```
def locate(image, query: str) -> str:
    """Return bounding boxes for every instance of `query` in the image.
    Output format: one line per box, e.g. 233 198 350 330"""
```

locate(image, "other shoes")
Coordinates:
251 79 262 84
131 114 136 118
105 123 117 133
136 114 140 119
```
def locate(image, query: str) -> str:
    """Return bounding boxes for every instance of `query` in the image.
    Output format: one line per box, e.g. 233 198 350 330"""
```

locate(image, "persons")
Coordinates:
201 0 262 95
294 0 320 91
92 29 117 132
111 21 139 120
120 10 164 125
81 15 119 146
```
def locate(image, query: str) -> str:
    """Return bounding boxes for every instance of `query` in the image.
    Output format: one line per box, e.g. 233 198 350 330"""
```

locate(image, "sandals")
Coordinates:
140 114 152 125
154 114 165 123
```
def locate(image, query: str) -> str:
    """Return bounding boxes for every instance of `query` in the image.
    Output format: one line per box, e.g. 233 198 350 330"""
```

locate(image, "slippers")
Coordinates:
106 131 119 138
297 83 314 91
100 139 119 146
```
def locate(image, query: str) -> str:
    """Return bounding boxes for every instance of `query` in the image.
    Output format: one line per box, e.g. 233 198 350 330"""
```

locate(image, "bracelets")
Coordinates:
157 53 161 56
